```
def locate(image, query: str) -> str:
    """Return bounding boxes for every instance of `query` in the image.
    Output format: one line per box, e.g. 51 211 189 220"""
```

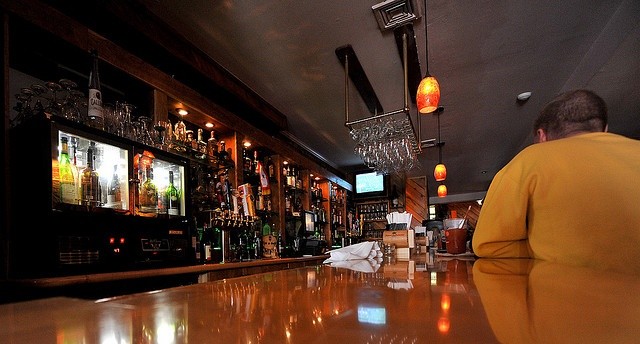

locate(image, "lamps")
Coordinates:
451 203 458 217
416 0 440 113
437 142 448 197
433 107 446 182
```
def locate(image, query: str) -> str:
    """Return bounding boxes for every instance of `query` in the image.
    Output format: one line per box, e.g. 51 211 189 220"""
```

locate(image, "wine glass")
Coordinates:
105 97 155 148
349 119 416 173
12 80 87 124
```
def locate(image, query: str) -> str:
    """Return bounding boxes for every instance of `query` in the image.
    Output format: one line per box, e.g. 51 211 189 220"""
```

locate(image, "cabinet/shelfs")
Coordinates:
153 69 237 209
285 144 310 231
237 118 283 229
331 177 347 239
359 197 389 231
312 162 332 239
334 23 427 176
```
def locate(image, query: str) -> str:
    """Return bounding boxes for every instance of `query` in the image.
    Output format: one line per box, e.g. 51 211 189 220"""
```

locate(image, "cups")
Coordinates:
445 228 467 254
382 242 397 255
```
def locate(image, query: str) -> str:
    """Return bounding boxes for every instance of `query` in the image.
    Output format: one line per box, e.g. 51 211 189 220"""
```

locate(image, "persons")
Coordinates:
473 87 639 276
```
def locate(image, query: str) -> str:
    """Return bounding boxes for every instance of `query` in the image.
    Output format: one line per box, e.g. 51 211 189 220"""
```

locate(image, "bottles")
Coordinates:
174 121 186 141
313 226 320 240
266 156 274 178
139 164 157 213
272 223 277 240
196 129 207 153
60 137 81 205
359 204 387 220
265 188 272 211
252 232 259 259
291 168 296 187
208 132 218 156
297 171 302 189
243 147 251 171
52 147 60 209
278 234 282 259
311 199 326 224
331 208 342 226
108 164 121 205
254 151 262 174
241 230 252 261
352 215 356 229
256 186 265 210
166 171 180 215
311 182 323 198
331 184 345 201
201 231 213 262
285 190 295 216
287 167 291 184
218 141 230 162
89 65 104 131
167 120 173 146
81 141 101 207
262 222 270 236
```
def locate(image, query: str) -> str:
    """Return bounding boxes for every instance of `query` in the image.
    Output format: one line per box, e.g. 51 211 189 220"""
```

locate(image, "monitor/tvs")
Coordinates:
356 304 388 327
354 170 386 195
